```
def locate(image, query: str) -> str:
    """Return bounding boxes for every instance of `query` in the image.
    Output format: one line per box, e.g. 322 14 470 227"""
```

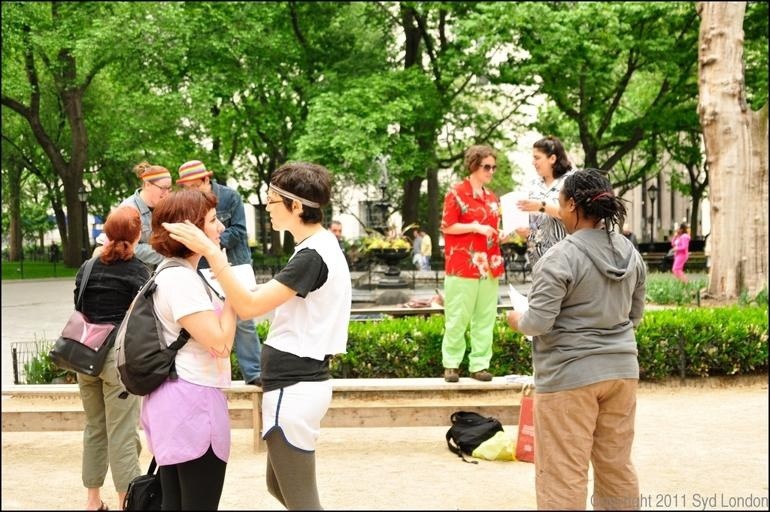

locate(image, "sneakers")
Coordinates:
470 369 492 381
444 368 460 383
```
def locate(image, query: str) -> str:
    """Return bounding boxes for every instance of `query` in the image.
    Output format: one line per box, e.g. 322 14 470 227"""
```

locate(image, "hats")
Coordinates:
175 158 215 185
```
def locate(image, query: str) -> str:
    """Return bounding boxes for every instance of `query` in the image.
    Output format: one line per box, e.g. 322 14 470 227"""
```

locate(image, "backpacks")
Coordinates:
113 260 213 396
445 410 504 465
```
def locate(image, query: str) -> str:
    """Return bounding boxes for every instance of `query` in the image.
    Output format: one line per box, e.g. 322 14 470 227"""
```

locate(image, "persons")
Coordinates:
50 240 59 263
705 234 712 273
622 223 639 252
139 188 237 512
118 161 173 276
328 221 353 272
419 228 432 269
440 145 504 382
670 223 691 284
515 136 578 272
176 159 263 385
506 168 647 511
72 205 152 511
161 161 352 510
412 228 423 269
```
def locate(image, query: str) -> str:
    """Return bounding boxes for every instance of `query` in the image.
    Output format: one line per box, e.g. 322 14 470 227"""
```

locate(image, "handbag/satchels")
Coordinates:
121 456 161 511
48 308 117 378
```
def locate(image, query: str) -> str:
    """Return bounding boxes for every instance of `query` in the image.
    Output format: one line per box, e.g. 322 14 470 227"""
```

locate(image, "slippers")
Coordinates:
97 500 108 511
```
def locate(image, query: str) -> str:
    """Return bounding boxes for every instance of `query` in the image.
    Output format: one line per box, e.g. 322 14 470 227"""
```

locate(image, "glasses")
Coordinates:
149 180 173 193
266 195 294 205
478 163 497 172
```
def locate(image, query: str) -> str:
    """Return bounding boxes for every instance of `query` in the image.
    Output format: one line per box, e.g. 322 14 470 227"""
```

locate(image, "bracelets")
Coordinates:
209 262 232 280
539 201 545 212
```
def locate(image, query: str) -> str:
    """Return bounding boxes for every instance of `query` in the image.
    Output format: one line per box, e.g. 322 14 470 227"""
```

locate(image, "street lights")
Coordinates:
647 181 660 250
378 177 388 226
